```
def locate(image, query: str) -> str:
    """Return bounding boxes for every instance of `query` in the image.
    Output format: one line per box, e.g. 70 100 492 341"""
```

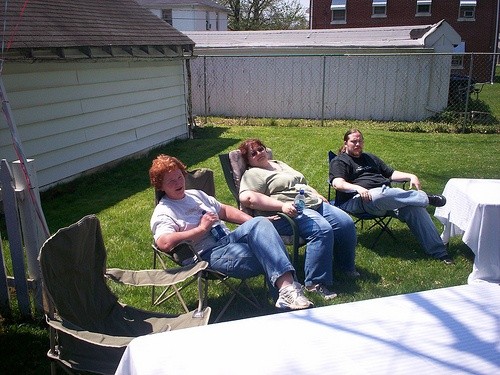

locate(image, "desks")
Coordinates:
114 283 500 375
433 177 500 286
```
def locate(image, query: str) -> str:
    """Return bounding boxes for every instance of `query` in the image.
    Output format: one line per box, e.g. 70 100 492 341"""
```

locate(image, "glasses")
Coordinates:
247 146 264 156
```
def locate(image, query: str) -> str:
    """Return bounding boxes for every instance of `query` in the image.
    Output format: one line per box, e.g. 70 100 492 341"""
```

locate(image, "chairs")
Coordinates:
216 148 306 270
35 212 211 375
146 166 263 326
328 150 408 246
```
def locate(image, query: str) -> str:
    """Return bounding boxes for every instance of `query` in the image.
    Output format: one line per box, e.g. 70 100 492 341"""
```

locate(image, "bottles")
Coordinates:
294 189 306 218
202 210 230 246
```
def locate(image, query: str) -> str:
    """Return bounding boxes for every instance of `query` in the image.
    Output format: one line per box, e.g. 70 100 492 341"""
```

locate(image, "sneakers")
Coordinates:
439 255 452 265
305 283 337 301
344 271 360 279
423 190 446 207
275 281 314 310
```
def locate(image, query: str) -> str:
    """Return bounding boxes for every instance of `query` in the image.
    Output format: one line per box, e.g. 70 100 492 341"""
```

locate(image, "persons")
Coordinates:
328 128 454 264
149 154 315 310
238 139 360 300
